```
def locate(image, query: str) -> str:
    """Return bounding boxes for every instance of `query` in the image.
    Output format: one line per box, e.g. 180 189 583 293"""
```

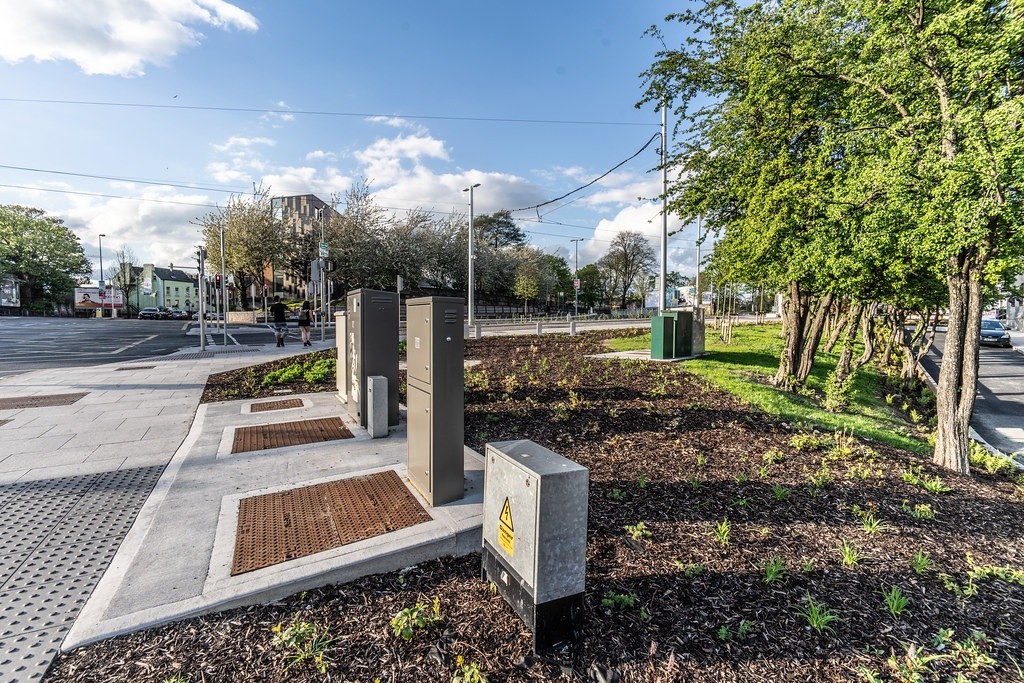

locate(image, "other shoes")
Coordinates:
304 343 307 346
307 341 311 346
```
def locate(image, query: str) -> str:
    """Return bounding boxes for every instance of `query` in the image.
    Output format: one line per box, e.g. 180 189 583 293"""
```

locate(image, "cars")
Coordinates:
206 313 217 320
192 312 199 320
173 310 188 320
980 319 1011 347
158 306 173 320
138 307 161 320
219 313 224 320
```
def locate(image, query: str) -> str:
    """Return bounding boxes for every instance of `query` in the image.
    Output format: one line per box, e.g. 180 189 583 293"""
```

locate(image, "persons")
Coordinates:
270 295 295 348
298 301 315 347
81 294 97 303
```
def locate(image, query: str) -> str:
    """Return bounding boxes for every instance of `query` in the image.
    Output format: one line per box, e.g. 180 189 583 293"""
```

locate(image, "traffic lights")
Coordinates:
216 275 220 289
263 286 269 297
197 249 204 276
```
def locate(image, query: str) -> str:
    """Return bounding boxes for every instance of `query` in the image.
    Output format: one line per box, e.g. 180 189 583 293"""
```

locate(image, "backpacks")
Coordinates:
299 309 309 320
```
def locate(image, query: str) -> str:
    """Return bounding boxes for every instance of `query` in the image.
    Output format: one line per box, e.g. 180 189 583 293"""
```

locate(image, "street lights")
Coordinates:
570 238 585 316
462 184 482 326
695 213 702 313
319 206 327 327
99 234 106 317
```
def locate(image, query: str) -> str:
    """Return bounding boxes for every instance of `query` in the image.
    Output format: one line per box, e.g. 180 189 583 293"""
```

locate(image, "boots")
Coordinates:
277 339 281 347
280 338 284 346
